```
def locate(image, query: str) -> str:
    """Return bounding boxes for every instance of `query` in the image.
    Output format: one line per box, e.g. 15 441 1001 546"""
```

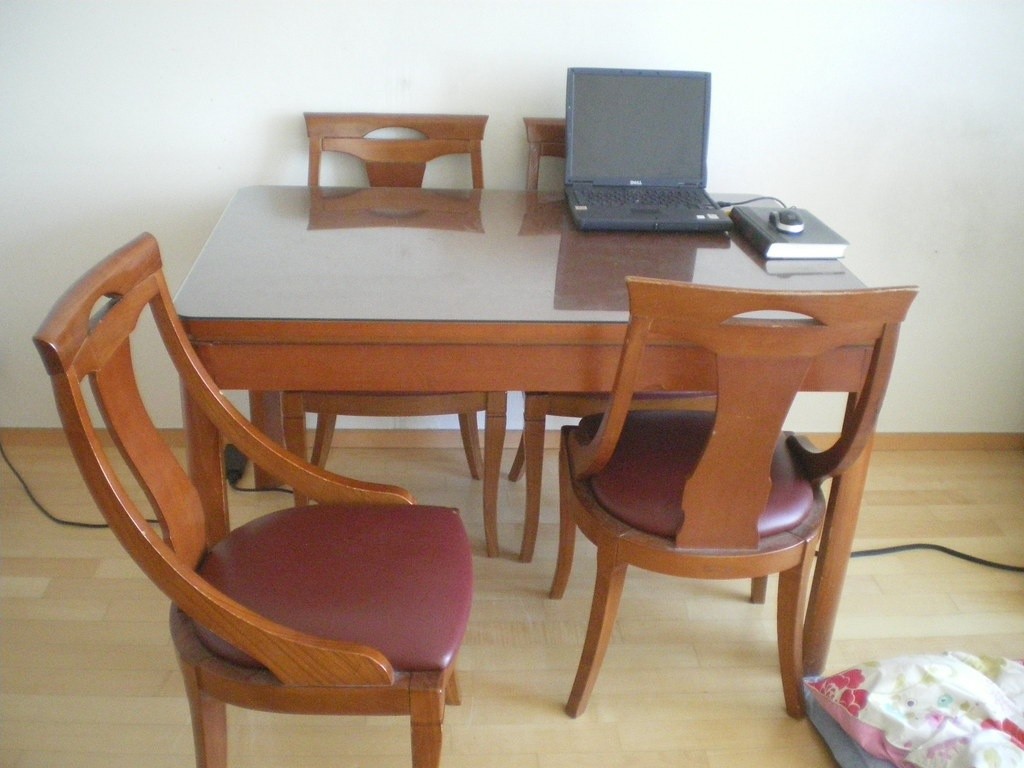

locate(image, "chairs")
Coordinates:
282 113 508 557
508 118 718 563
550 275 920 722
32 232 475 768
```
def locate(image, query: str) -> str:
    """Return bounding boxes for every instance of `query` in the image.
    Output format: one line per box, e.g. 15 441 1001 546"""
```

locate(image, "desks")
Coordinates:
175 184 874 676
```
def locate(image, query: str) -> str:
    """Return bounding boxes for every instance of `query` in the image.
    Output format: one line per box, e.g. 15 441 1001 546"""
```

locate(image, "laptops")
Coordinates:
563 67 734 231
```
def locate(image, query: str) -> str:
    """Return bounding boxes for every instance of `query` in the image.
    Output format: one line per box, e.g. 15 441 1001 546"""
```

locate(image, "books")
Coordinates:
728 229 846 275
729 206 850 259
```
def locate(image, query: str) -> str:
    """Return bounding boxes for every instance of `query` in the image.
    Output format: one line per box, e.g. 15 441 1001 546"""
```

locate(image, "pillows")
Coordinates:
808 653 1024 768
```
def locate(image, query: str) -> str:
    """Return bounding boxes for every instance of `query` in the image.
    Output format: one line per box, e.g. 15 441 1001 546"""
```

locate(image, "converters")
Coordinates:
225 444 246 479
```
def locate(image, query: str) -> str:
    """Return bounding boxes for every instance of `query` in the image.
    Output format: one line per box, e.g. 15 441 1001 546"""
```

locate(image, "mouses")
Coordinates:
770 208 805 233
